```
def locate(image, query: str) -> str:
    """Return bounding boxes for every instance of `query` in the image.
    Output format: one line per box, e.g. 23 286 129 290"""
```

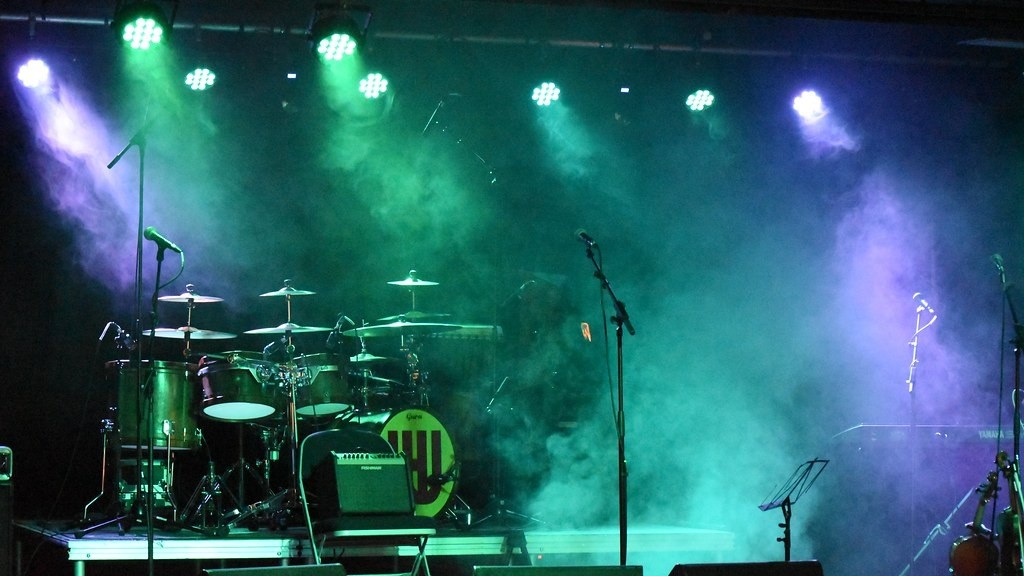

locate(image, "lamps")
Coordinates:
113 0 178 54
306 0 372 66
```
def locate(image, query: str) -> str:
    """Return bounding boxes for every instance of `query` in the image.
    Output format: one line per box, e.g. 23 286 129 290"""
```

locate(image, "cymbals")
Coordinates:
259 286 317 297
386 277 440 286
243 322 335 335
142 326 237 339
157 292 225 303
342 311 464 338
349 353 400 367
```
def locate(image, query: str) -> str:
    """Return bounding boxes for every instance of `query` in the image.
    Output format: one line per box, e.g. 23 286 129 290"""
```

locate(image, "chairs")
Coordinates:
299 429 436 576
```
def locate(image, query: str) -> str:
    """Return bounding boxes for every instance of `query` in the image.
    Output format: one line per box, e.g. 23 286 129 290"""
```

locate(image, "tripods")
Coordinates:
443 378 552 533
73 108 321 539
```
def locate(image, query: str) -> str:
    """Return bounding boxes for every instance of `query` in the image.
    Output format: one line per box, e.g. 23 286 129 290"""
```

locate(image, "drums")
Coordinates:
289 351 355 418
198 349 285 423
105 359 200 450
405 324 503 405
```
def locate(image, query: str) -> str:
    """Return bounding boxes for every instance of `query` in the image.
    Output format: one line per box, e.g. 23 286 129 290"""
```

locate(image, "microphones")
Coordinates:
912 293 937 317
263 336 289 355
989 254 1004 273
112 321 137 350
422 94 447 135
327 316 345 347
144 226 183 254
574 229 598 248
502 280 534 307
427 474 458 486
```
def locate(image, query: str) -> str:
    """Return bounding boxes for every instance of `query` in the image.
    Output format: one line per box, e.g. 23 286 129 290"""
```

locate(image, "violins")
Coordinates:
949 451 1024 576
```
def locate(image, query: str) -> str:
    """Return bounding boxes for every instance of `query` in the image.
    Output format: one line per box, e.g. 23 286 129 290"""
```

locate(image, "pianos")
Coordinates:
830 423 1024 576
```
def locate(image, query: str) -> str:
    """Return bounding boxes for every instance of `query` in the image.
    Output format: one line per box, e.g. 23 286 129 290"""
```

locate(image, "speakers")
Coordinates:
307 451 416 517
0 481 16 576
203 563 346 576
470 565 644 576
666 560 824 576
